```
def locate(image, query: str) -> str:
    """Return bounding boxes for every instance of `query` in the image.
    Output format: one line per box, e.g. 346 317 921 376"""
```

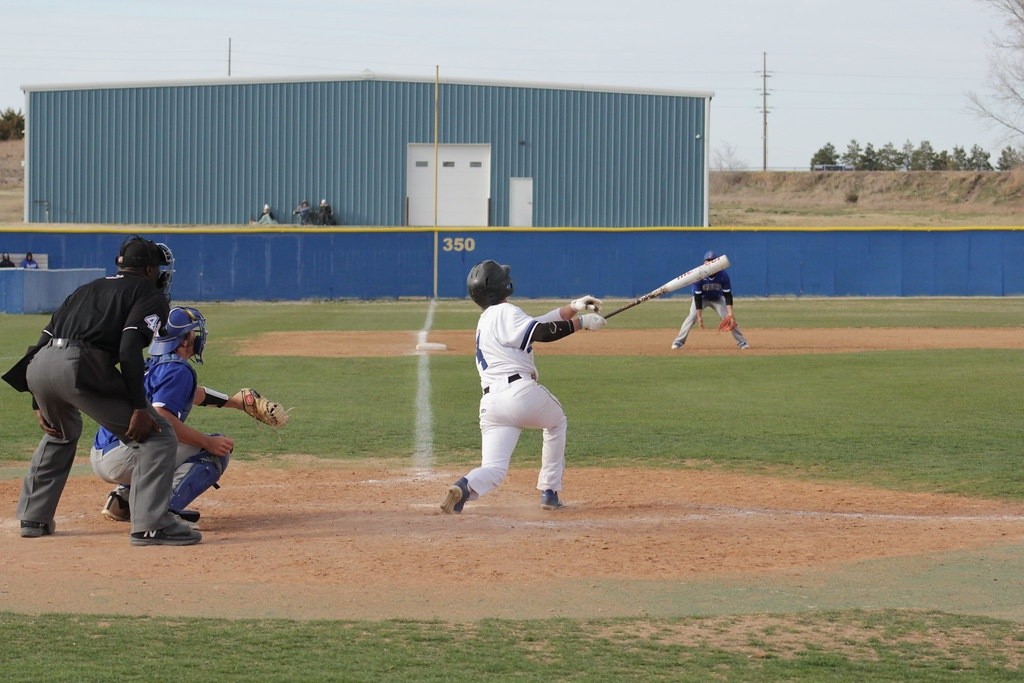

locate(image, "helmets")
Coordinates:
466 260 514 307
149 306 208 366
115 234 176 304
704 251 717 260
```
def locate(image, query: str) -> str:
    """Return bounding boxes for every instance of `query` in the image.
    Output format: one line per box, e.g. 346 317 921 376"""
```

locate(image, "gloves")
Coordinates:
570 296 603 314
578 313 607 330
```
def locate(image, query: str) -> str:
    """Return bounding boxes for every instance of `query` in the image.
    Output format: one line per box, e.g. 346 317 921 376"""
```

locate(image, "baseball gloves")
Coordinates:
240 388 288 427
719 315 737 332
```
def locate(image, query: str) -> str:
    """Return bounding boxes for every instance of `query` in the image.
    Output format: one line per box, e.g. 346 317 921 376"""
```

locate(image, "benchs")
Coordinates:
0 253 48 269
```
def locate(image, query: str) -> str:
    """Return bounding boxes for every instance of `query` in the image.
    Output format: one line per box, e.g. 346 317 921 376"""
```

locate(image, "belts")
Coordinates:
51 338 109 350
101 440 122 456
483 374 521 394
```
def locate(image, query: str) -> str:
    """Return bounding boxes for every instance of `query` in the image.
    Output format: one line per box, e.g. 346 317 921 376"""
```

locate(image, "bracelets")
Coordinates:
578 316 583 329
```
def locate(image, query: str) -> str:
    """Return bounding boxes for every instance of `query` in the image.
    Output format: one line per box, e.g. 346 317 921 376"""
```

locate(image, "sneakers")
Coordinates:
440 477 471 514
541 489 564 509
671 341 682 349
101 490 131 522
168 512 200 529
130 519 202 546
20 519 55 537
737 341 750 350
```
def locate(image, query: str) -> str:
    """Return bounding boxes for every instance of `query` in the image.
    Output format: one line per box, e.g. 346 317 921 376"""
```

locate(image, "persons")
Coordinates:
319 199 331 225
19 235 204 545
671 250 751 350
89 306 252 522
439 260 608 512
297 200 312 224
260 204 274 225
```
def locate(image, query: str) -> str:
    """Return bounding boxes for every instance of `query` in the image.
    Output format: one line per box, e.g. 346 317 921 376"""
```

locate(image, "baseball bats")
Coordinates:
602 255 732 319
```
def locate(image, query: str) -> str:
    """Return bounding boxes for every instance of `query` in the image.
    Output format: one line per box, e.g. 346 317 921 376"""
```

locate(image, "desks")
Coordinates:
0 268 106 315
